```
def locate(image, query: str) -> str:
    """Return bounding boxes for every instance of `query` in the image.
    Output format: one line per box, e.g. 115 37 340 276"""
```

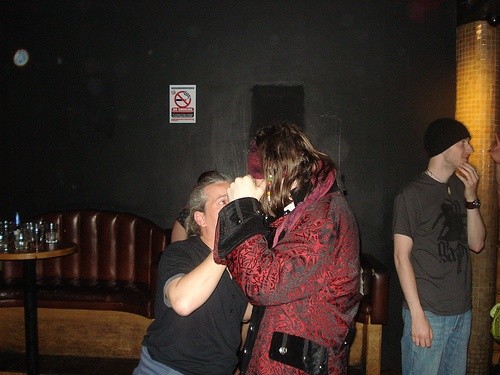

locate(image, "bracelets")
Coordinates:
465 198 481 209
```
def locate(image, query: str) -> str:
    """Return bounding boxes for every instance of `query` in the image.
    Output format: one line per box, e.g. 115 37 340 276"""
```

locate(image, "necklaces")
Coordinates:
426 169 445 184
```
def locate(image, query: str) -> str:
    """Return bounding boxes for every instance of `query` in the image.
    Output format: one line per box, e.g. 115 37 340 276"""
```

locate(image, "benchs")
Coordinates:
0 210 391 375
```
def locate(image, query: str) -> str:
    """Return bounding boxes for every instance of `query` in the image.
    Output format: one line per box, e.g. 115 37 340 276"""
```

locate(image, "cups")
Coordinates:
0 220 59 251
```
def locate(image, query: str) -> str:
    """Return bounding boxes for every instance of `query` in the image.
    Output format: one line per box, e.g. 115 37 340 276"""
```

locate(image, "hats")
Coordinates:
424 118 471 159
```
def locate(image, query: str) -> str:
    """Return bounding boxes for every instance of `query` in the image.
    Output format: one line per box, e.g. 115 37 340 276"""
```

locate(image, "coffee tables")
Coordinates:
0 239 79 375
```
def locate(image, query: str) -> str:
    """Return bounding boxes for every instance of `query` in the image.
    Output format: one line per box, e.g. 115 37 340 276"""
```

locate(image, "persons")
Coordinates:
390 118 500 375
213 118 363 375
130 170 253 375
